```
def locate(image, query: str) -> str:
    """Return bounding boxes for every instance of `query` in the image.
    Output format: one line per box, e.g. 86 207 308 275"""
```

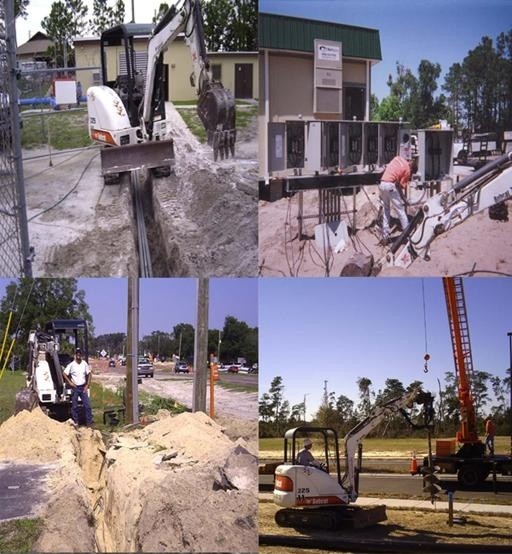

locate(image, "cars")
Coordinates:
101 355 127 369
227 365 239 374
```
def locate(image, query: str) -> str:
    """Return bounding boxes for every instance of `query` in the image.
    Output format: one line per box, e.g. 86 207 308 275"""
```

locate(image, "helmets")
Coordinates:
303 438 314 446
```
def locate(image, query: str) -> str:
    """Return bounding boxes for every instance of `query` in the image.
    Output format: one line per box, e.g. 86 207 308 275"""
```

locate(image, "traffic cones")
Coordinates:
409 450 418 472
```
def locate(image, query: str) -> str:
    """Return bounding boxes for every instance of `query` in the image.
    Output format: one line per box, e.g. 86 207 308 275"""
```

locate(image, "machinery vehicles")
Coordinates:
26 317 92 423
85 0 240 185
272 386 434 532
417 278 511 489
407 120 512 164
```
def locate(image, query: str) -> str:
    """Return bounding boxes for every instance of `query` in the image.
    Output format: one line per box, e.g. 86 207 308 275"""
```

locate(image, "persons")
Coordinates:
484 417 496 456
63 347 108 425
297 439 321 468
379 156 419 243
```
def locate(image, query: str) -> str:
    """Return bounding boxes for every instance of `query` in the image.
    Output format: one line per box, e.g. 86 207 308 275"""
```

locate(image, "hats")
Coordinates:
75 347 82 354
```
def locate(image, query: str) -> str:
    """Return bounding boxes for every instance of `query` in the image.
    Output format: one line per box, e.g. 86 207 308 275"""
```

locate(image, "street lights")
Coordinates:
218 322 231 361
176 322 183 360
151 332 160 354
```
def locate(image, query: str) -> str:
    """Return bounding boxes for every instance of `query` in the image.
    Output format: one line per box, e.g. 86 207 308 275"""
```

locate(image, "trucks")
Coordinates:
19 59 54 82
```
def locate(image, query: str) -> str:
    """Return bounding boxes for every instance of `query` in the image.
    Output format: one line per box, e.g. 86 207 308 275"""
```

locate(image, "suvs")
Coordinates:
137 357 154 377
174 360 189 374
